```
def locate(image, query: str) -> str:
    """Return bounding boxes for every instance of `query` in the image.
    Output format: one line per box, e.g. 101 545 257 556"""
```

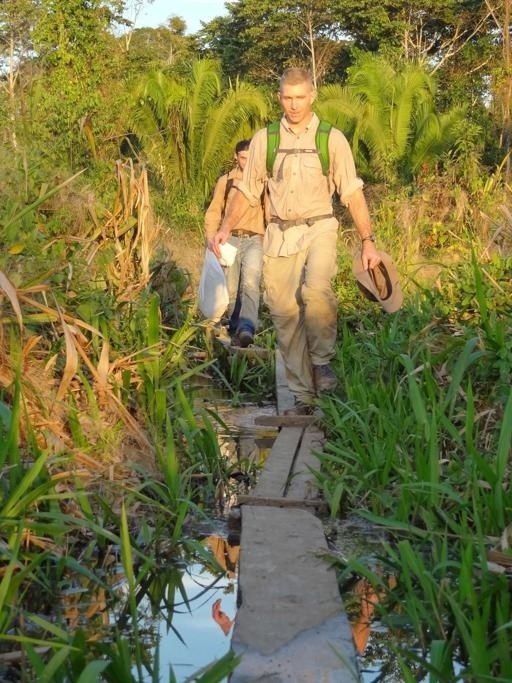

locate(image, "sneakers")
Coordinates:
314 366 337 392
239 330 254 348
284 403 312 417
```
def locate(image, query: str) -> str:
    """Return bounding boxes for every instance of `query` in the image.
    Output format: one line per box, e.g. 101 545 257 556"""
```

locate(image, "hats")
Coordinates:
353 251 403 313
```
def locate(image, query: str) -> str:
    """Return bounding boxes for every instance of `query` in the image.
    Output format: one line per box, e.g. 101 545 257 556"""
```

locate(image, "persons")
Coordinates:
211 592 378 657
214 68 380 415
205 139 270 346
208 534 240 577
